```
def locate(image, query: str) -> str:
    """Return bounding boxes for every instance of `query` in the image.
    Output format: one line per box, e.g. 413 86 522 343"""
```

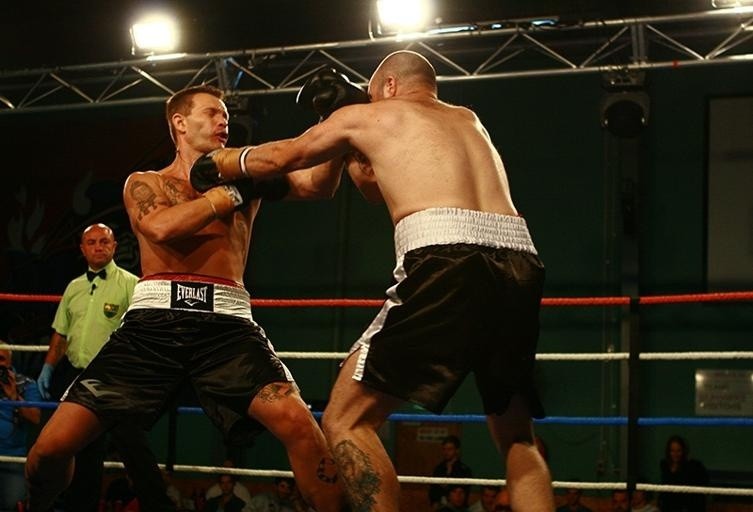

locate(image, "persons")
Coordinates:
9 86 359 510
0 340 42 509
189 50 558 510
37 223 158 510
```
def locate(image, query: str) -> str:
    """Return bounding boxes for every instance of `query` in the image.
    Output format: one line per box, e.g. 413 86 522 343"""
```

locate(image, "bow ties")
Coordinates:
86 269 107 283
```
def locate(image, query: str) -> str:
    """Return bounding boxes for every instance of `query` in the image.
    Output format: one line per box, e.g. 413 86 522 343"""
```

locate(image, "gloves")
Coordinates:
296 69 372 121
37 364 53 400
189 145 292 218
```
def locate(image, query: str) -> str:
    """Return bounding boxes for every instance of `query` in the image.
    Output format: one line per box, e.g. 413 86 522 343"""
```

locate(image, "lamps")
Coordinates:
600 69 657 139
221 97 257 147
